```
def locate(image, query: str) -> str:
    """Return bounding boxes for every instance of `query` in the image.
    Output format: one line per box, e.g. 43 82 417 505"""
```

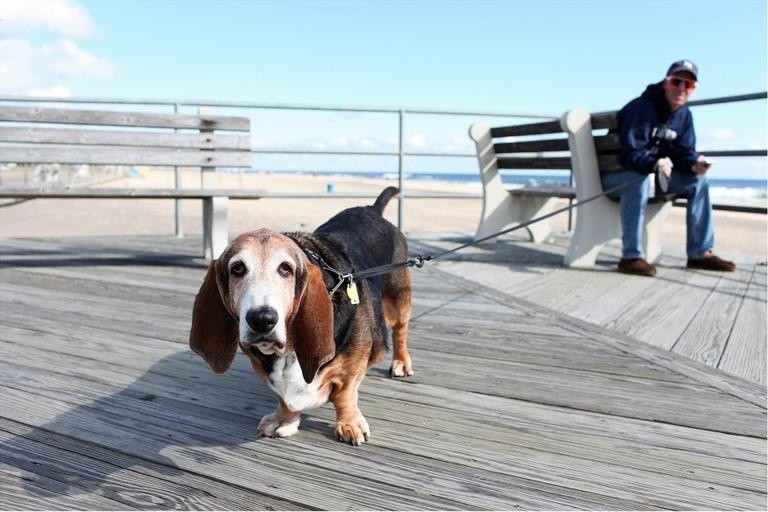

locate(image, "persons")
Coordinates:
600 58 736 277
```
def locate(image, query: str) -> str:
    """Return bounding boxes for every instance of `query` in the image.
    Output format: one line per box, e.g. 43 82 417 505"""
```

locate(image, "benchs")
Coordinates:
468 110 670 270
0 105 265 259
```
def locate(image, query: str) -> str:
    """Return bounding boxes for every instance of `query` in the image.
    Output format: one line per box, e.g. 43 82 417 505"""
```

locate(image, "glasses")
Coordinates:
666 78 696 88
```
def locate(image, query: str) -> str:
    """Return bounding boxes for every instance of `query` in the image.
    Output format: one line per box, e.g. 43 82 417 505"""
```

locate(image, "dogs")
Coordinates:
188 186 417 447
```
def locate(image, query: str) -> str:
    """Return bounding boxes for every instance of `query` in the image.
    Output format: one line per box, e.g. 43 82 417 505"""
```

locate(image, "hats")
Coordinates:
667 59 698 81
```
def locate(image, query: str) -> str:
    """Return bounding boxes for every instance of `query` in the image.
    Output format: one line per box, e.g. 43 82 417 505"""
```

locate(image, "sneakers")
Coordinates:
618 257 657 276
686 254 736 271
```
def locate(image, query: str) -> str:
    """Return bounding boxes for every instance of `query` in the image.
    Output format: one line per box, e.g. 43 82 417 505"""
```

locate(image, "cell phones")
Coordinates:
700 161 712 167
